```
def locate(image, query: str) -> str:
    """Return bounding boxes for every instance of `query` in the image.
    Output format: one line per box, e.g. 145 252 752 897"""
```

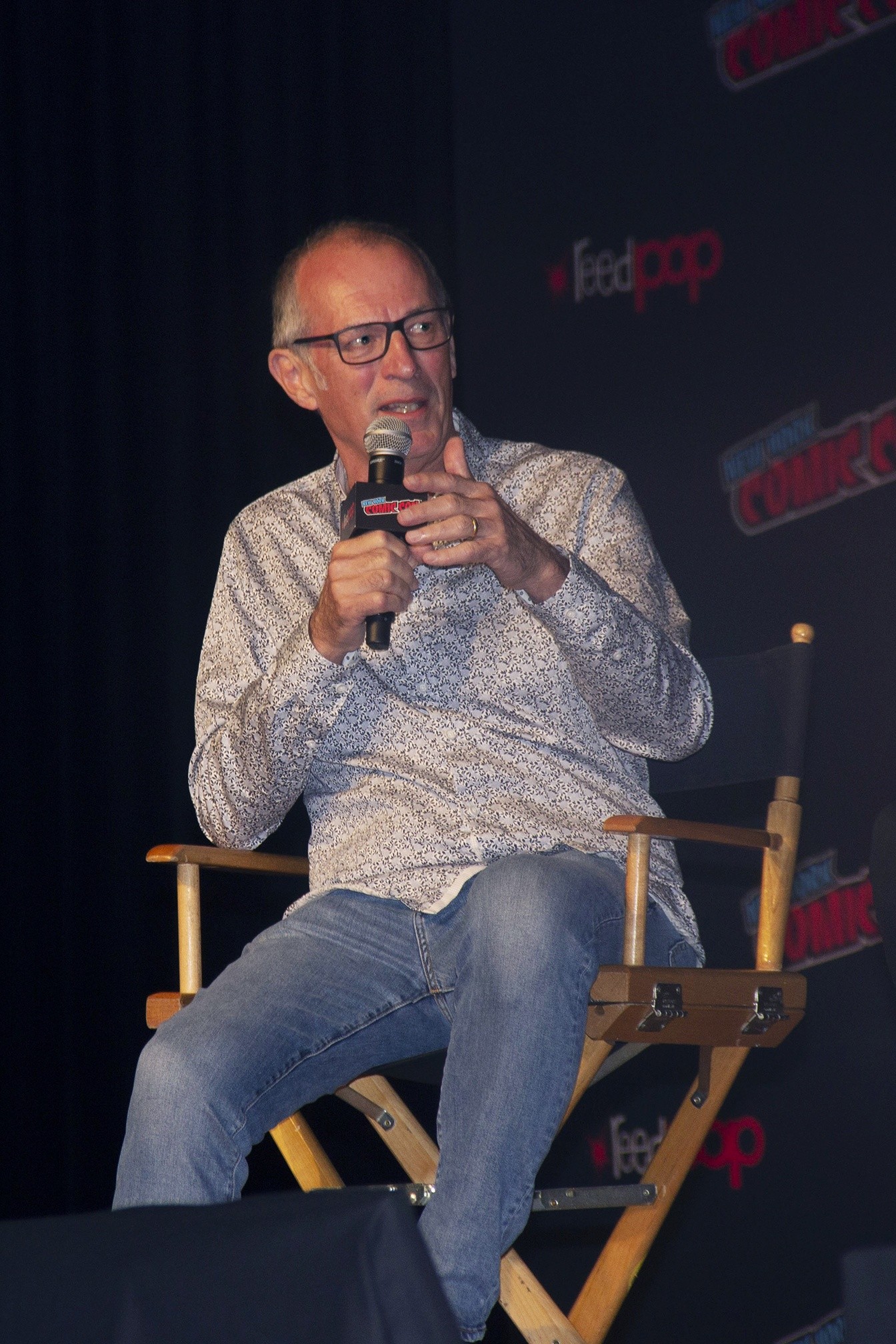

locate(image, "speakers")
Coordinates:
0 1187 463 1344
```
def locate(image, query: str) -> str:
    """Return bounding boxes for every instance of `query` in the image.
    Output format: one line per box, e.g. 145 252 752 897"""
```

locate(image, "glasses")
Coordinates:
294 305 457 365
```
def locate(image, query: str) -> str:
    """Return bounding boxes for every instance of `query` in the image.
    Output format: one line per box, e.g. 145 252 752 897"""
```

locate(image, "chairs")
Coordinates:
147 621 814 1344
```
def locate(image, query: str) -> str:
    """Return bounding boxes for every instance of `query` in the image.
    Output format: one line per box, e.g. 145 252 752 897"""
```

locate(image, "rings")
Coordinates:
471 517 479 540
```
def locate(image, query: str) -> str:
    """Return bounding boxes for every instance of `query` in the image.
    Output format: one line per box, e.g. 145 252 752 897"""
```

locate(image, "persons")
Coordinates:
111 223 718 1343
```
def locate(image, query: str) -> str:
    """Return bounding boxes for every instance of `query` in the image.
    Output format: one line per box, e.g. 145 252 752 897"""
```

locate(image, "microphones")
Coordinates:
339 416 427 650
